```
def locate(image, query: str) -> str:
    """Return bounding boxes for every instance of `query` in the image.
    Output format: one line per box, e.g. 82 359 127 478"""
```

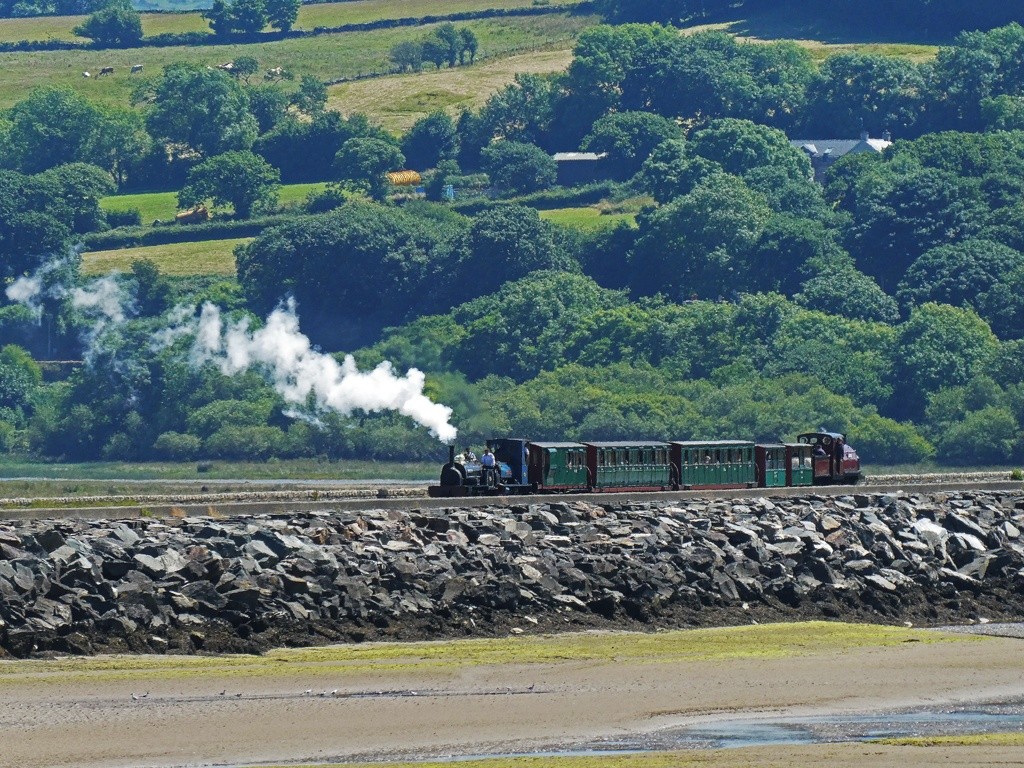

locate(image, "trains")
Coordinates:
425 428 865 499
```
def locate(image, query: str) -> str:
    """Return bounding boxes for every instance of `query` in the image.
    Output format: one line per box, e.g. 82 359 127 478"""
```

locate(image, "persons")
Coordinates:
481 449 495 468
815 445 826 455
835 438 844 474
464 448 476 462
